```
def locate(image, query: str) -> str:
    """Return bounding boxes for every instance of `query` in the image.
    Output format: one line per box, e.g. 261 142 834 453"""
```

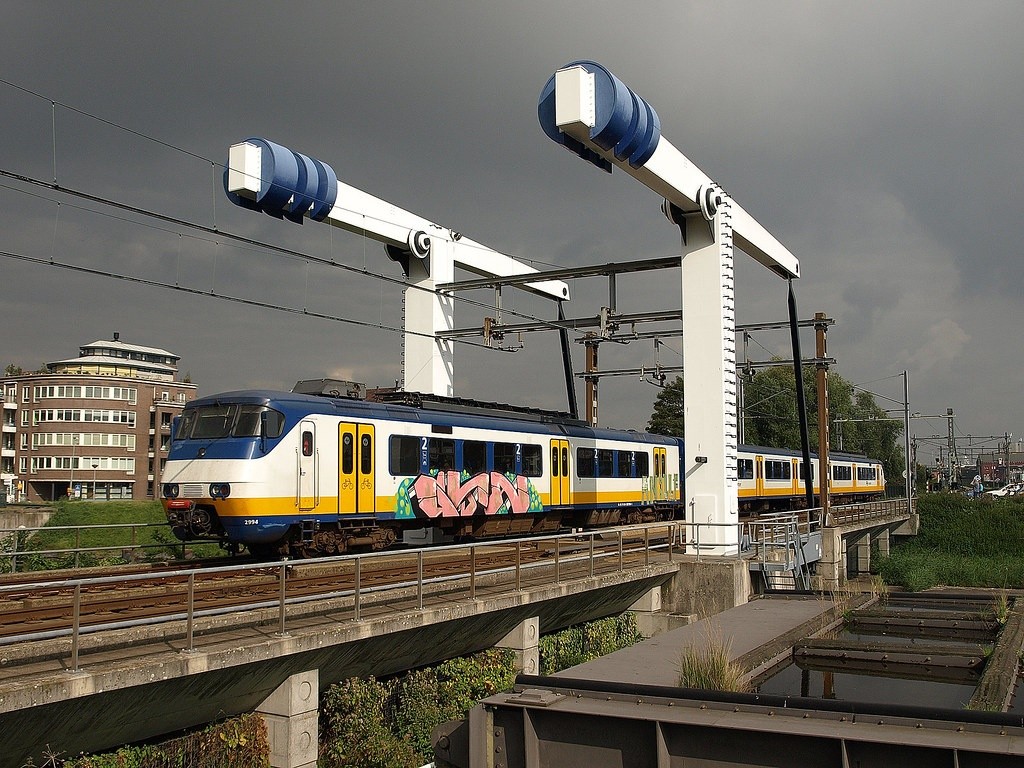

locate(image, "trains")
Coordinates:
158 377 887 562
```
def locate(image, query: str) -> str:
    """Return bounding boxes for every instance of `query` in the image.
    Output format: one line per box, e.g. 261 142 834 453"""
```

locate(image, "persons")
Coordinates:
979 481 983 493
971 480 979 496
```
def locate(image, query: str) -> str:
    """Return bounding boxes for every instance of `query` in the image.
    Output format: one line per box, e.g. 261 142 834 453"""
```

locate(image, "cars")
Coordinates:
984 483 1024 499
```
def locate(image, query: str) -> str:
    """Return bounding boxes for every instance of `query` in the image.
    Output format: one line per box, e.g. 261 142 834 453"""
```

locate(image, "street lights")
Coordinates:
92 463 99 500
